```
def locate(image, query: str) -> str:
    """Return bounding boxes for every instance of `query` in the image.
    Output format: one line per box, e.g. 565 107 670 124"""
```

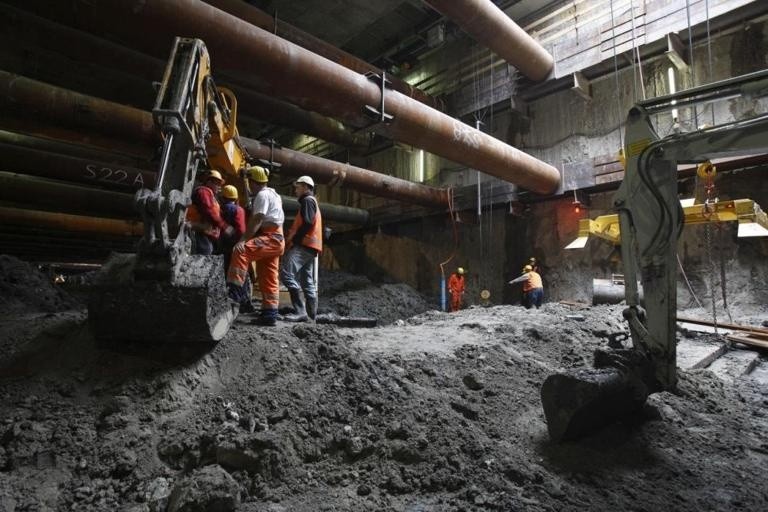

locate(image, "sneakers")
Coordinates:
250 315 276 325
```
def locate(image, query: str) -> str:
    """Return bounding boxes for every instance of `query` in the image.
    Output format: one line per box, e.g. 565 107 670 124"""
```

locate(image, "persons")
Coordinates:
226 166 286 327
278 175 323 321
446 266 464 313
188 169 231 254
507 264 543 309
221 183 253 312
520 257 542 306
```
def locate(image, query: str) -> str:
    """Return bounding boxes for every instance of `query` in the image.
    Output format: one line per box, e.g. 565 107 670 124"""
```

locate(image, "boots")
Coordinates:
306 298 318 324
284 291 309 322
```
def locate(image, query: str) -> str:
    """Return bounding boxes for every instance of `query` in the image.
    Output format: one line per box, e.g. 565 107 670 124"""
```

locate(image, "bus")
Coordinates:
40 263 103 288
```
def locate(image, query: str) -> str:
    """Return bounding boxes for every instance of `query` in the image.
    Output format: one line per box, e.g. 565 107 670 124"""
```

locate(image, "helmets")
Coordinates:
248 165 269 183
525 265 532 270
457 267 464 275
222 184 238 199
292 175 315 188
202 169 225 185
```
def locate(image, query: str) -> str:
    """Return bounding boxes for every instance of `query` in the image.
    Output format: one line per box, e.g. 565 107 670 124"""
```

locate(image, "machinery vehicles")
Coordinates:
81 36 306 345
541 69 768 461
